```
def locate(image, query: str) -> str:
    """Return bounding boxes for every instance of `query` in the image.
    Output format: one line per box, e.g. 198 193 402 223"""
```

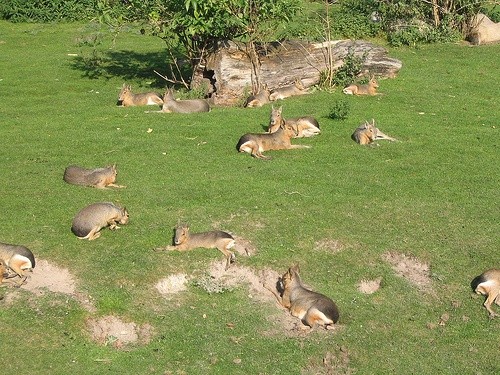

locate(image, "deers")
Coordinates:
242 82 275 109
268 78 312 102
279 261 339 334
63 161 127 191
70 203 130 242
144 84 213 115
342 74 384 98
236 119 312 159
153 224 236 271
470 267 500 318
266 102 322 139
116 84 164 108
350 118 402 148
0 242 36 288
